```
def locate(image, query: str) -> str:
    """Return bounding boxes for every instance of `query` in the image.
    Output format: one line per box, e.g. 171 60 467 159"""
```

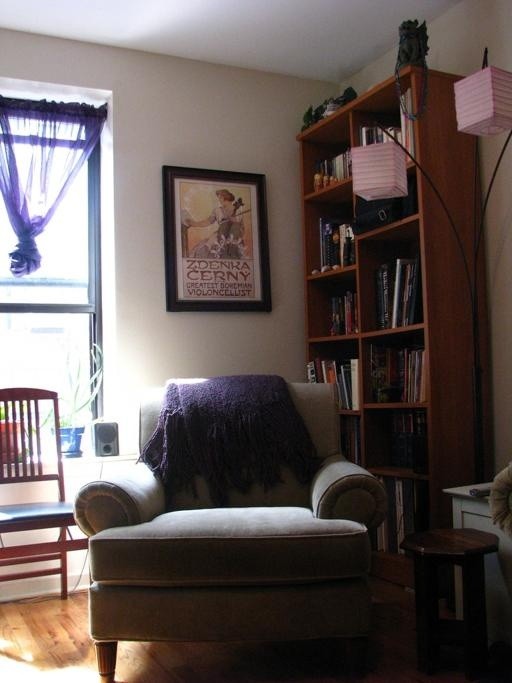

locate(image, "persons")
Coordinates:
185 189 244 259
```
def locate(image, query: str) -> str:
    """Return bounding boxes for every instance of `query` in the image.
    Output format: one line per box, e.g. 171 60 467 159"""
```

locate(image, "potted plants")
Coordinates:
0 395 86 464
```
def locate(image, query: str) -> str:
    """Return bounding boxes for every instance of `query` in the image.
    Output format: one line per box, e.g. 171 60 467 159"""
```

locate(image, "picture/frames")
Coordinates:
161 163 272 313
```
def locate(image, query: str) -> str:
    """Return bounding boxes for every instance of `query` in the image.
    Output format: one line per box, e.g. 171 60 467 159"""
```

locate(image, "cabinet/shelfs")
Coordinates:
295 64 496 590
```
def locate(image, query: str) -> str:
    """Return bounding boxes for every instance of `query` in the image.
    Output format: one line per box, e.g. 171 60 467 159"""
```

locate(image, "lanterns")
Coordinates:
351 142 408 202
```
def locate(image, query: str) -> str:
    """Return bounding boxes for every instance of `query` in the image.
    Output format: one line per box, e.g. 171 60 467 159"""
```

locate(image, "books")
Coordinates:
315 146 352 188
389 410 426 475
368 474 426 555
353 172 419 234
335 414 361 466
320 217 356 272
307 355 360 410
364 343 426 403
374 249 422 330
358 88 415 162
329 290 359 337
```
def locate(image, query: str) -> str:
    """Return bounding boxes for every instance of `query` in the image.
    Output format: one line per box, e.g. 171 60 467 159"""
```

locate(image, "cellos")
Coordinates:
206 197 245 259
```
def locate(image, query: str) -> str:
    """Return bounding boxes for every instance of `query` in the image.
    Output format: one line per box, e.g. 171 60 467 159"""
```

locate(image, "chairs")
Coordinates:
0 387 89 600
74 383 390 682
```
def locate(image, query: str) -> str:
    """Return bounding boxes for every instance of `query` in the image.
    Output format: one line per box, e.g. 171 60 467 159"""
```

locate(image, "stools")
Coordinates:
399 527 499 681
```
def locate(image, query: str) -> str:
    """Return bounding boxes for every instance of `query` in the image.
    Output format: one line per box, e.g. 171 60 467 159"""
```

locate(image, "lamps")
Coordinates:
343 46 512 487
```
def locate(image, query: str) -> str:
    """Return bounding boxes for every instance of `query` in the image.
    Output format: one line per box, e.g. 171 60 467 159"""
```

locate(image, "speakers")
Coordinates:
94 423 119 456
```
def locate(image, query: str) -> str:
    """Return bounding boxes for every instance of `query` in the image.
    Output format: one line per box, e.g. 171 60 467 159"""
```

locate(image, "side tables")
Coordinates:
441 479 511 645
